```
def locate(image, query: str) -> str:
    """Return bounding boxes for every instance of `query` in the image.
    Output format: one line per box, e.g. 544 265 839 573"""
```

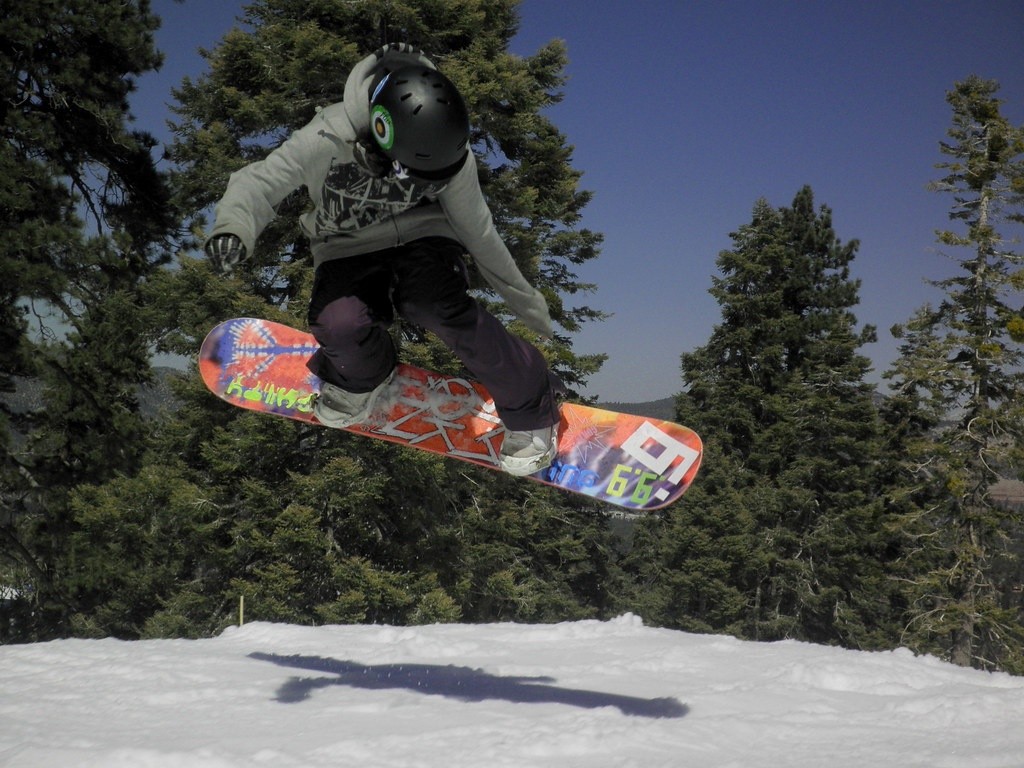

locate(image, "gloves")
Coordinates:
207 234 247 275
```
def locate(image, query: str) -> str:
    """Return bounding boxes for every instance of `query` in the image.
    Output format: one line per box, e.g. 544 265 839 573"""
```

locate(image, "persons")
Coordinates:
203 43 561 478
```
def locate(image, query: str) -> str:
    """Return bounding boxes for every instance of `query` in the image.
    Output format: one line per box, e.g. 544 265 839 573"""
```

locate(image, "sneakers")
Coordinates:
501 421 560 477
317 364 393 429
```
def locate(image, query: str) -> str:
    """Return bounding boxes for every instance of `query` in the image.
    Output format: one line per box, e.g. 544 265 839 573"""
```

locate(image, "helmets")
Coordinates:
369 64 470 183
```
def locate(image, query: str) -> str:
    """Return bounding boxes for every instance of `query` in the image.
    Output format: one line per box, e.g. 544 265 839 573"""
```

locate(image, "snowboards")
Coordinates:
196 316 705 512
